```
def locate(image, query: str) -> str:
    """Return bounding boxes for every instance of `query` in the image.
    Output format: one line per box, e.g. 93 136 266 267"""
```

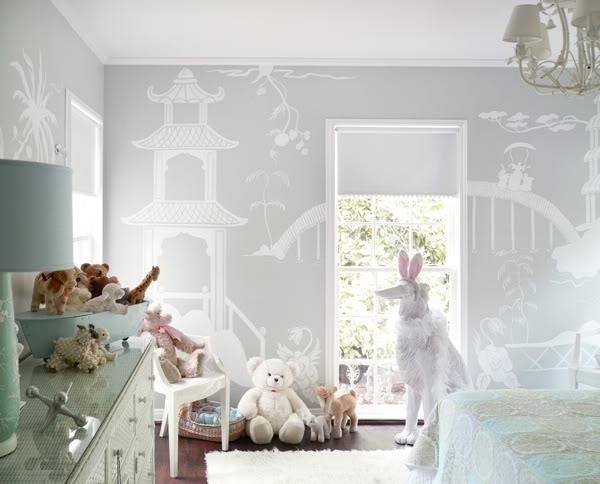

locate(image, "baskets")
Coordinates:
179 402 247 444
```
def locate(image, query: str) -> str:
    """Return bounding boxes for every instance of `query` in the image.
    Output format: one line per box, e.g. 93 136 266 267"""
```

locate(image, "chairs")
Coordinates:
154 334 231 478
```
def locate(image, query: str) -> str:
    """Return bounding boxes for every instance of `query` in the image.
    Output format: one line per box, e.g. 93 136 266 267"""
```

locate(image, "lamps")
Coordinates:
0 159 74 457
502 0 600 97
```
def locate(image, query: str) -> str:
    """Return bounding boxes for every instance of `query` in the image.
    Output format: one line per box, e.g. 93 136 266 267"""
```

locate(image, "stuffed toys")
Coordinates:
375 249 467 449
305 414 331 443
235 355 313 445
29 263 209 381
315 382 359 438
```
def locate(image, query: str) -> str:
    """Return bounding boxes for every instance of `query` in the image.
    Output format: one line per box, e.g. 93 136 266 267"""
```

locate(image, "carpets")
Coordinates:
203 446 433 484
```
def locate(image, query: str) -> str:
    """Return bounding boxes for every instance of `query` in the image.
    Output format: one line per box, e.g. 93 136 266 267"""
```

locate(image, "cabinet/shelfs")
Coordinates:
0 336 155 484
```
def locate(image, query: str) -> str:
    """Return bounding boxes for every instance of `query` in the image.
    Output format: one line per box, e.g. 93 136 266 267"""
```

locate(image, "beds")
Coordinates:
405 388 600 484
